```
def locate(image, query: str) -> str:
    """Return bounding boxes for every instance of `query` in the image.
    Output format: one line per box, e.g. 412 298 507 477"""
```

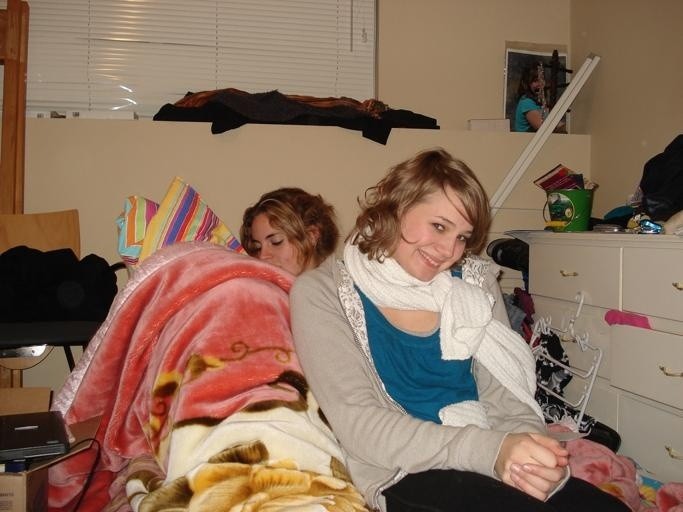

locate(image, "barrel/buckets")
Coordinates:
542 189 596 233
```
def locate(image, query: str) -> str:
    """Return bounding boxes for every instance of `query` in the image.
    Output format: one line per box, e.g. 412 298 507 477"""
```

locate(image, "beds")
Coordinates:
23 117 683 512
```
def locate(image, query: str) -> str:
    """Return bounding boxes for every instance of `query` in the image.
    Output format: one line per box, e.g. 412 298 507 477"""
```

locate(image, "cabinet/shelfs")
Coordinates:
503 230 683 484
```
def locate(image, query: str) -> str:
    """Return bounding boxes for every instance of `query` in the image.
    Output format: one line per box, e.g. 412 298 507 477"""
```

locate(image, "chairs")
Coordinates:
0 209 119 373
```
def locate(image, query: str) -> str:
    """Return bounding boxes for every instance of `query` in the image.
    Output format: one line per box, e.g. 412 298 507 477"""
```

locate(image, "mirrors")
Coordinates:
0 344 54 370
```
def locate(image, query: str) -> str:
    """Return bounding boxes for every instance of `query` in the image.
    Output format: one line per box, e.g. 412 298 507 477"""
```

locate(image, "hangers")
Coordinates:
528 291 603 432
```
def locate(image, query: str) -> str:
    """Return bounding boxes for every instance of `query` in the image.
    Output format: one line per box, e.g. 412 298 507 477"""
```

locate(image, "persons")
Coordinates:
289 147 633 512
515 66 565 133
239 187 340 278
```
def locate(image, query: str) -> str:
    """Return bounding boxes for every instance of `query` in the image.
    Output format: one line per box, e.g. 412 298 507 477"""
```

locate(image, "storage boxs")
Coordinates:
0 387 103 512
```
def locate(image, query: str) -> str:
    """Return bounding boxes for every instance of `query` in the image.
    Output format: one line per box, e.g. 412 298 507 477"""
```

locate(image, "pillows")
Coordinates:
113 194 161 271
134 176 249 269
